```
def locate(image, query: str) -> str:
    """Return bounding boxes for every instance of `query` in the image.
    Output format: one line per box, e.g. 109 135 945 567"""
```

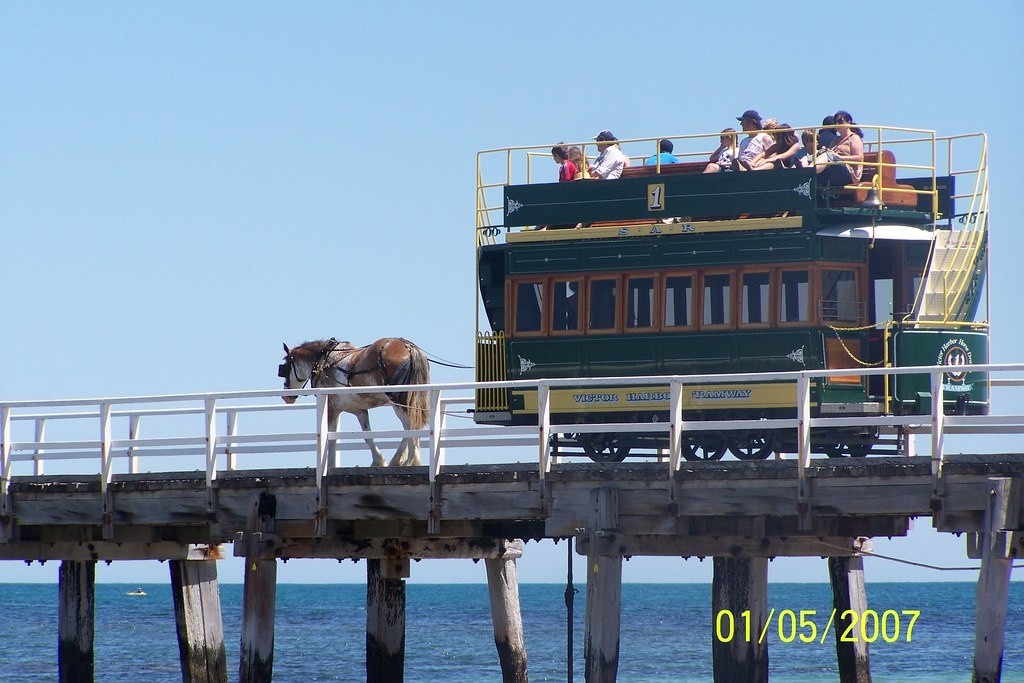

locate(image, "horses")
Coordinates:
277 336 432 466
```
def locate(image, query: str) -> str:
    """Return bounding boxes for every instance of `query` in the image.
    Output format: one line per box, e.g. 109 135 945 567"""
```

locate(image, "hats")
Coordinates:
655 139 673 152
736 110 762 120
592 130 616 140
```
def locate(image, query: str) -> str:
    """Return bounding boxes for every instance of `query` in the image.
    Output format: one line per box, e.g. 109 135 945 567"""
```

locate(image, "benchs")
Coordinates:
545 150 917 230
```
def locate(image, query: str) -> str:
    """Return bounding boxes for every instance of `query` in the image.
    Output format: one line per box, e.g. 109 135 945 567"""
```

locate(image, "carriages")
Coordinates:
277 124 993 467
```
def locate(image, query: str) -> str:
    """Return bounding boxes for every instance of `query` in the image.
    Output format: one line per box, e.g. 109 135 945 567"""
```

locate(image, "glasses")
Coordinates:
805 138 814 142
596 139 605 142
834 118 844 124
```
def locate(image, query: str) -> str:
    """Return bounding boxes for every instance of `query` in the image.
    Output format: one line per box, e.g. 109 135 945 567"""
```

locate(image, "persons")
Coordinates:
645 140 678 165
551 130 630 182
702 110 864 185
